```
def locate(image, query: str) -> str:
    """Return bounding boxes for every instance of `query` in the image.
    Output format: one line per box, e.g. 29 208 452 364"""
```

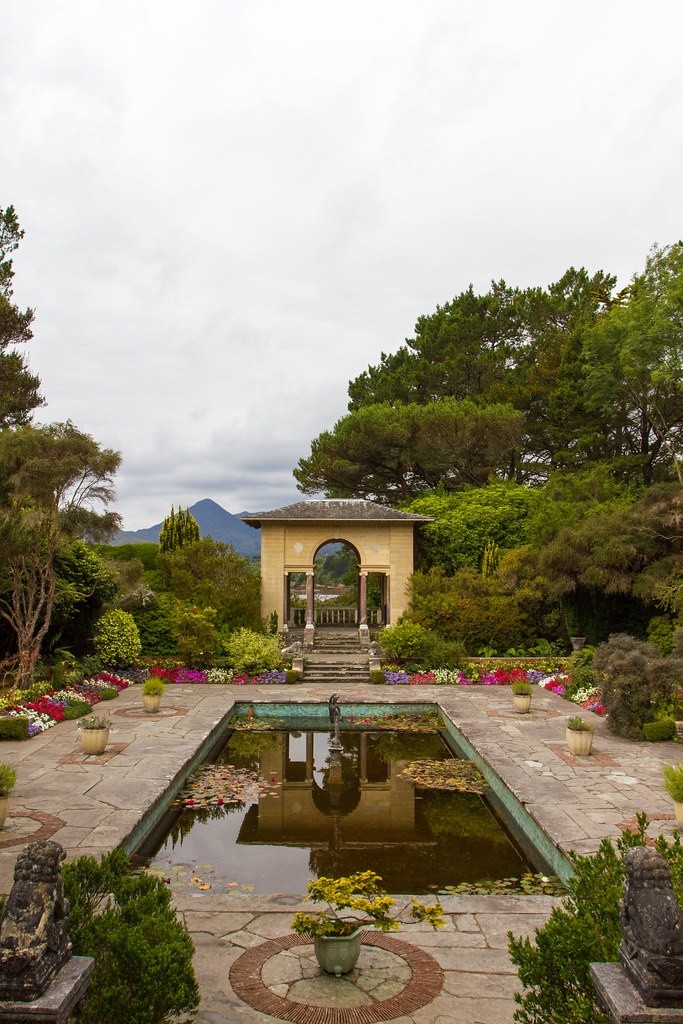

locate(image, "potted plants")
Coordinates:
662 762 683 827
565 716 592 756
0 761 17 830
142 678 166 713
511 681 533 714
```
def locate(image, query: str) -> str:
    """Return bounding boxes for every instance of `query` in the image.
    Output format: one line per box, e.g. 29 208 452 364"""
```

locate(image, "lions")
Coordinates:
280 641 303 655
0 838 72 978
370 641 382 655
624 845 683 956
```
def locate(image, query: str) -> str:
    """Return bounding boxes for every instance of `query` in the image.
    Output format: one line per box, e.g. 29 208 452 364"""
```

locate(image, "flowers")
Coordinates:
76 715 112 729
292 870 446 937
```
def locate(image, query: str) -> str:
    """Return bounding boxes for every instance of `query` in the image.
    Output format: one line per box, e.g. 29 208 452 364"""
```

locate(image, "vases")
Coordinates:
314 925 364 977
81 729 110 754
